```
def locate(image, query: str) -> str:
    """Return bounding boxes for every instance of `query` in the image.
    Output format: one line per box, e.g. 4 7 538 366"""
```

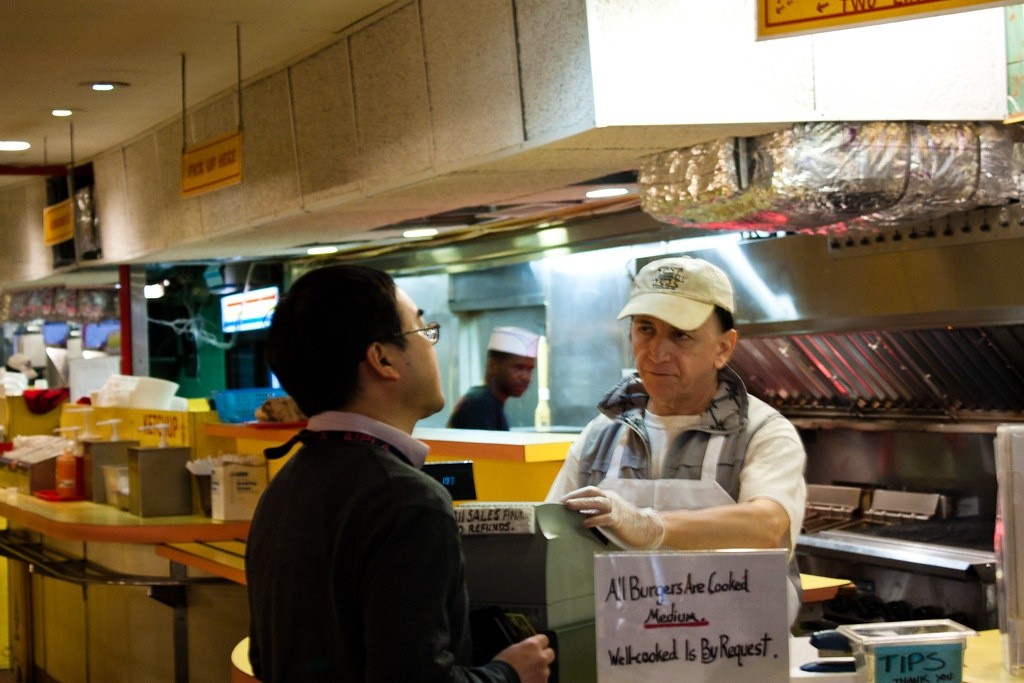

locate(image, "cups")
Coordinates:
195 474 211 517
102 464 128 504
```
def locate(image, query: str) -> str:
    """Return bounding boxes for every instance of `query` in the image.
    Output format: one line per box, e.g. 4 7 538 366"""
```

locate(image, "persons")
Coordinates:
447 326 540 432
245 262 554 683
542 254 807 629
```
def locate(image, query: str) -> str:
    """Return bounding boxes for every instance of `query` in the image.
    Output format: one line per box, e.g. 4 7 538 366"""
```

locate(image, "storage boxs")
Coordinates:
210 462 269 521
837 617 979 683
81 439 192 519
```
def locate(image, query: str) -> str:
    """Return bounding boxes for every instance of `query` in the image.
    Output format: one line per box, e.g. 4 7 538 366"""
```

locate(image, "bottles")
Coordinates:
56 442 94 501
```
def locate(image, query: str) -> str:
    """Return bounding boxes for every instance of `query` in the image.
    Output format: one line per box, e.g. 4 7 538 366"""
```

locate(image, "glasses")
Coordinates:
391 321 441 346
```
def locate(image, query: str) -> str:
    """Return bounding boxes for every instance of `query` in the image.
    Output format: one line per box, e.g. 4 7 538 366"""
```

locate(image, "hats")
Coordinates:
486 325 541 359
615 255 735 332
